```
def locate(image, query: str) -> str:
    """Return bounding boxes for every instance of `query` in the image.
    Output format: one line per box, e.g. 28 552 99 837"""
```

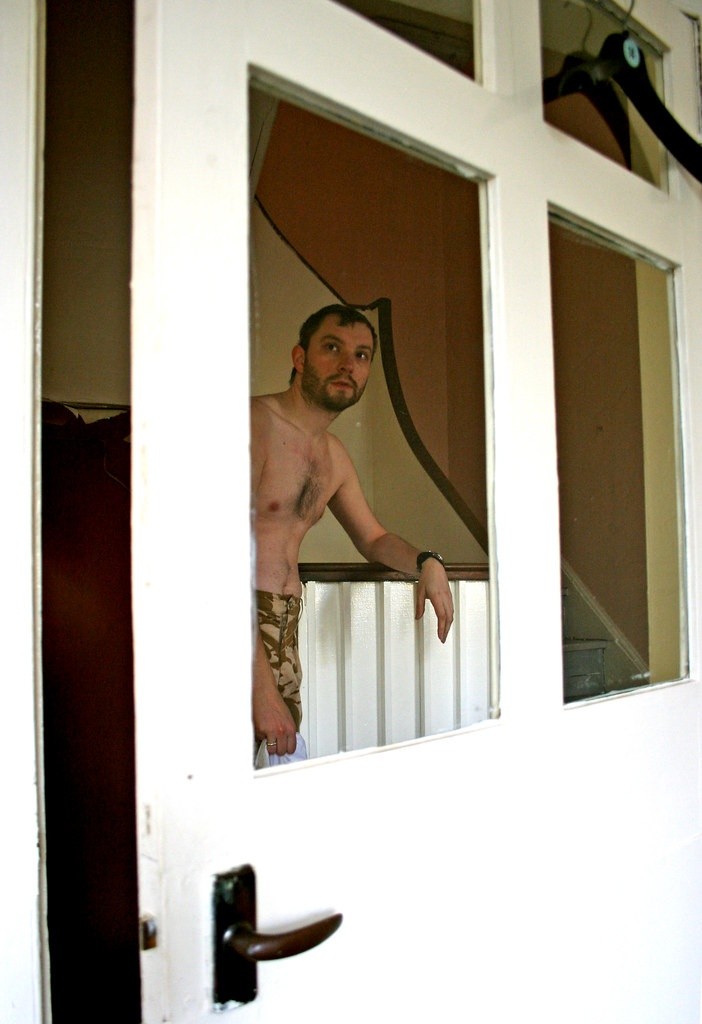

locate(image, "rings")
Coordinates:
266 742 276 746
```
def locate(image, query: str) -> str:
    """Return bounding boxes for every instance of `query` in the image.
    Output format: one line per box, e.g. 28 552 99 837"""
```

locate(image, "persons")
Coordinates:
250 304 454 768
41 401 140 1023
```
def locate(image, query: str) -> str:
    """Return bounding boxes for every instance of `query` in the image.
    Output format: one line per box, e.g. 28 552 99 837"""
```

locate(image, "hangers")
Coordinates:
560 0 702 184
541 0 629 169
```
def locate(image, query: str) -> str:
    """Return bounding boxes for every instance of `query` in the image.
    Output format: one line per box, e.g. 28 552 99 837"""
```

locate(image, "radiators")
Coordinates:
296 562 490 764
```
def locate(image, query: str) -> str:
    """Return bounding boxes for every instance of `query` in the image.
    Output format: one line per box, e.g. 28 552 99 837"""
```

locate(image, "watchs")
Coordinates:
417 551 445 572
